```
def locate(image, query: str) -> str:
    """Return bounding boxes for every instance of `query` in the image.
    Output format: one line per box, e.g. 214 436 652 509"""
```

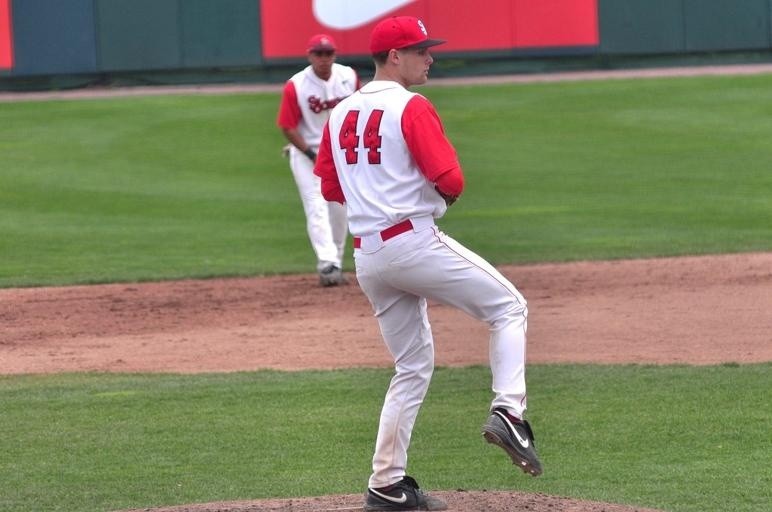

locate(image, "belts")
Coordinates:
353 218 413 249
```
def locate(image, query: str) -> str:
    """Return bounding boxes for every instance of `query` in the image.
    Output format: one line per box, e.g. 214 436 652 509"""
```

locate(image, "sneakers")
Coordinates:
363 474 448 512
319 265 342 285
480 407 543 477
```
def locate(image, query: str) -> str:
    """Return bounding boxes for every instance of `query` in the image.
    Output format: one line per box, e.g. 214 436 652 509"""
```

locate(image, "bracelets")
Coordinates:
305 148 316 161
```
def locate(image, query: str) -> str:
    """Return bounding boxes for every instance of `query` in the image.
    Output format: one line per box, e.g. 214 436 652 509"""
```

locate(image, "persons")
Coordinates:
313 15 542 511
277 33 361 287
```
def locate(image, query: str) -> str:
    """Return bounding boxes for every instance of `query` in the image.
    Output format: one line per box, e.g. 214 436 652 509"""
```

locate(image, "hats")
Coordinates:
368 14 449 57
305 33 338 55
